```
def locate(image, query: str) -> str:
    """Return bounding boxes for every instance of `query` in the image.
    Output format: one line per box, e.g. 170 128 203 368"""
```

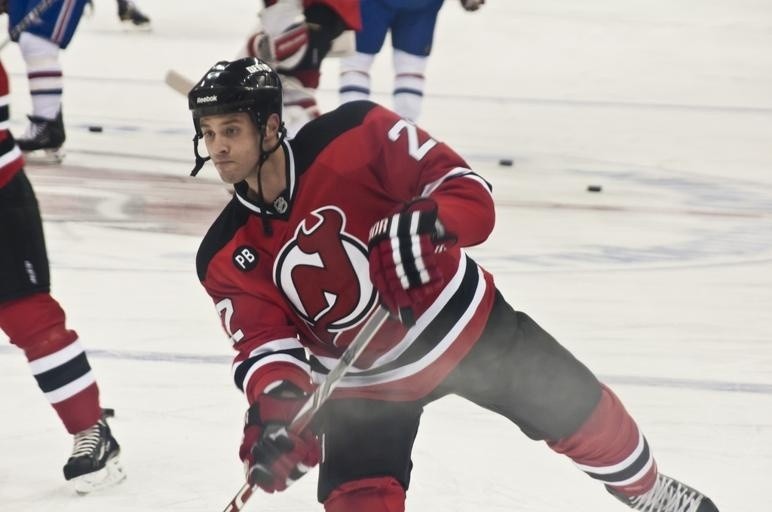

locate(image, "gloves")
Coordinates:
367 197 461 321
239 390 322 493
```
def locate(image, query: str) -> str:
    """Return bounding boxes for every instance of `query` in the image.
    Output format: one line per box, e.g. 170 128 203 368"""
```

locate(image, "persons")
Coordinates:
188 59 721 512
10 0 87 150
339 0 485 122
0 64 120 481
117 0 148 24
245 0 361 119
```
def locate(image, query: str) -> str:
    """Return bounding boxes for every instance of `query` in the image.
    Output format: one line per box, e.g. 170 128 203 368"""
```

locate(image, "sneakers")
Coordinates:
62 416 119 479
606 472 718 512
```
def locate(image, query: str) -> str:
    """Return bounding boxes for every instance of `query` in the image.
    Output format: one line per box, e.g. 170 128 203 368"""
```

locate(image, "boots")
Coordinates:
13 101 65 150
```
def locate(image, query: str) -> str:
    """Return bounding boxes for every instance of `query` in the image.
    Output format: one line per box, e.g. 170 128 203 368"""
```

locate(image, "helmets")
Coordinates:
187 57 282 134
